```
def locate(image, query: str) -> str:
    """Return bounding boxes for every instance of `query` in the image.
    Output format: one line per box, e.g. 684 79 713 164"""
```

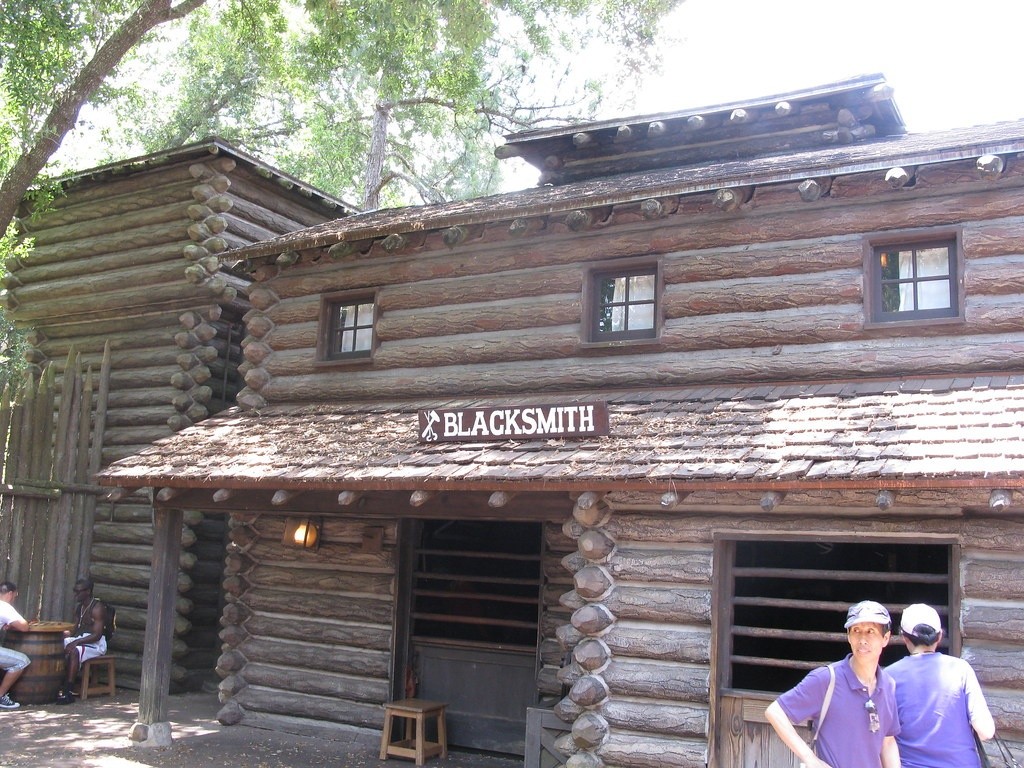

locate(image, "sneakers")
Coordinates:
0 691 20 708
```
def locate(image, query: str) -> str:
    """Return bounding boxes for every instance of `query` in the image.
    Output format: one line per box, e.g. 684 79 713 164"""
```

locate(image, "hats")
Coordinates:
844 600 892 628
901 603 942 637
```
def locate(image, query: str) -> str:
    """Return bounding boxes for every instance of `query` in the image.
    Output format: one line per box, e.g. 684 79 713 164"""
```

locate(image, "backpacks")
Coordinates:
76 600 119 641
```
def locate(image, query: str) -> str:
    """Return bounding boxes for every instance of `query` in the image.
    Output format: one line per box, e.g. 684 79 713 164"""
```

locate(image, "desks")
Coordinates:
1 621 76 706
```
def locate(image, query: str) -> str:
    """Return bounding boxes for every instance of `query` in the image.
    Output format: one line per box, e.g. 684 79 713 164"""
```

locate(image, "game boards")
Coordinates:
28 620 55 626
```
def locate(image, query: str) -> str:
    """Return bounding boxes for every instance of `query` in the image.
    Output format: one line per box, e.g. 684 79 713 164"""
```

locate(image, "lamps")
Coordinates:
293 517 324 551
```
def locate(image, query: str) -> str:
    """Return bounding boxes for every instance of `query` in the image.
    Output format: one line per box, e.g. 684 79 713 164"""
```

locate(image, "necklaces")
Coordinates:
77 596 95 629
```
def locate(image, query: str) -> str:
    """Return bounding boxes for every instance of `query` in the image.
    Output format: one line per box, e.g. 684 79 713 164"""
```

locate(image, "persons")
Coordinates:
0 581 30 709
881 602 995 768
56 578 107 705
764 600 903 768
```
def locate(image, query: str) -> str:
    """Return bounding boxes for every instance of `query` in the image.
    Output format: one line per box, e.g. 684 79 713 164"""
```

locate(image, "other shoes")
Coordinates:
56 689 79 703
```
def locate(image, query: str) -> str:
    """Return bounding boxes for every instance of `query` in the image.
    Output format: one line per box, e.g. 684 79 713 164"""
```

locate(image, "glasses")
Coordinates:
864 699 877 713
73 587 89 593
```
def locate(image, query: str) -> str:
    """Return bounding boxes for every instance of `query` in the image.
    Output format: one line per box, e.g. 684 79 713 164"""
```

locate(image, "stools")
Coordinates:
71 653 118 702
379 698 450 767
